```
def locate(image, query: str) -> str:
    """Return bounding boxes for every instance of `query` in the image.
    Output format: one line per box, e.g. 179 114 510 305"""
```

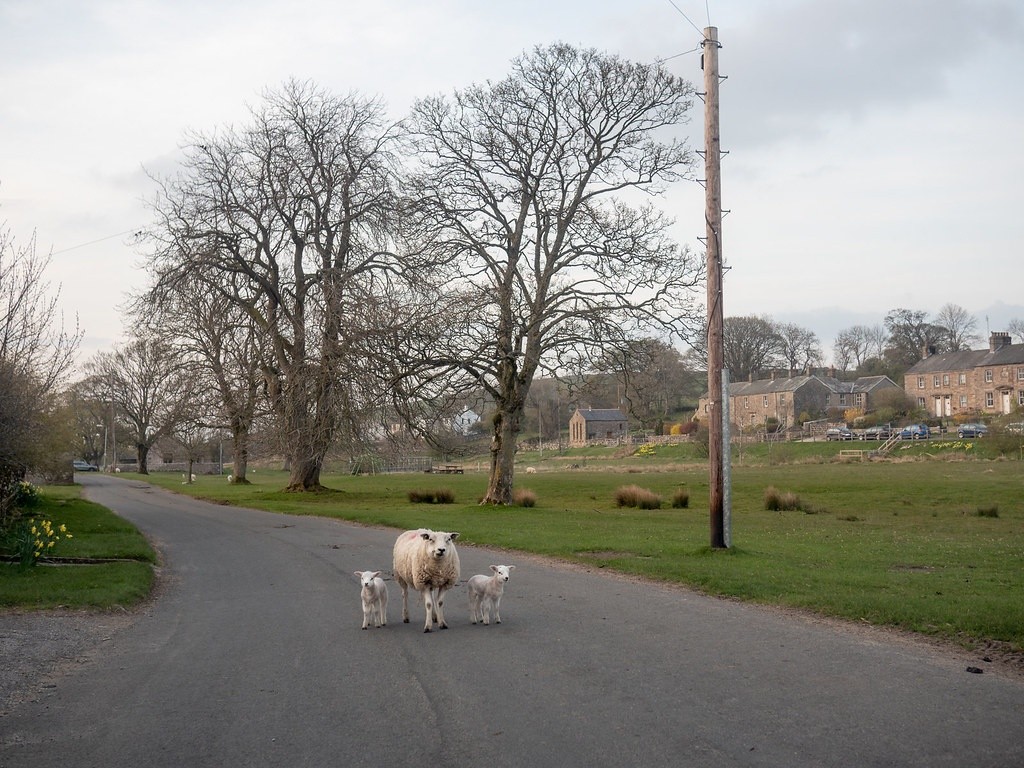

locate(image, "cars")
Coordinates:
1005 422 1024 438
73 456 99 472
857 425 895 441
825 426 856 441
957 423 992 439
897 422 931 440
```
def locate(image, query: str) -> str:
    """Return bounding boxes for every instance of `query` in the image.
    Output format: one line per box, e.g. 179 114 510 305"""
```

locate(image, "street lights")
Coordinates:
96 424 108 472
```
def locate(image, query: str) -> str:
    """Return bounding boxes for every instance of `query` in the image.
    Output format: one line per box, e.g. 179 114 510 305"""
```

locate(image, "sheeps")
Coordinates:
116 467 120 473
354 571 389 630
393 529 460 633
467 565 515 625
191 473 196 481
228 475 232 482
526 467 536 474
